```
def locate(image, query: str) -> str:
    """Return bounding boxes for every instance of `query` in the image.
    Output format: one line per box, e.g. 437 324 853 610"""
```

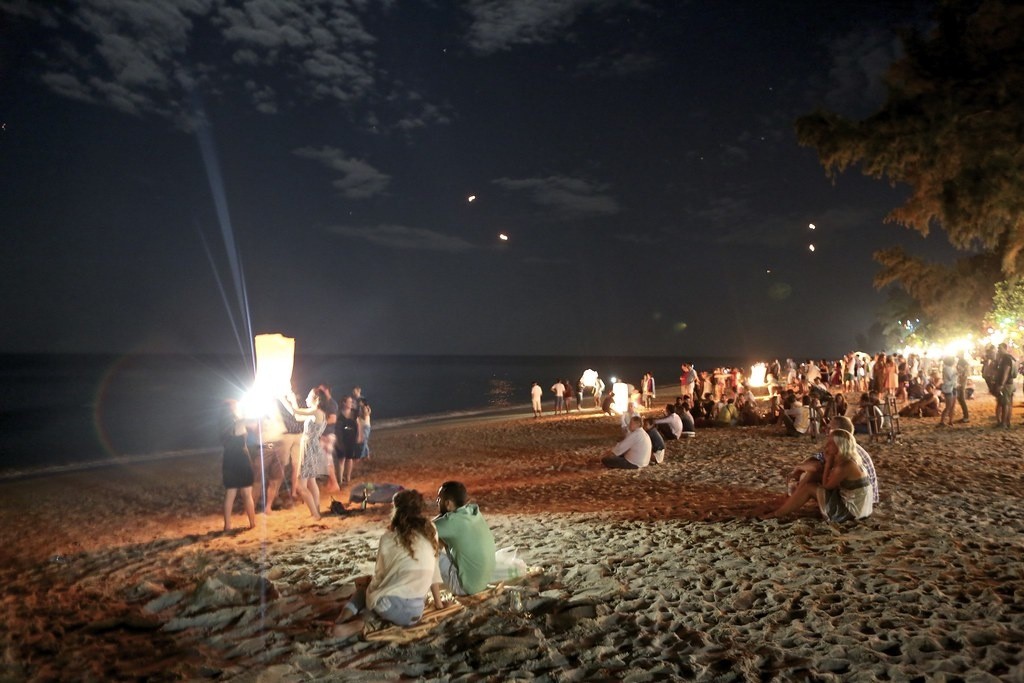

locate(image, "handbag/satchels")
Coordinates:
492 544 526 583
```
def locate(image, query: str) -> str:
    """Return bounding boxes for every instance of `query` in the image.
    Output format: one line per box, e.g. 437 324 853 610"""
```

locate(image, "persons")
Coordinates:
657 343 1024 527
576 379 585 410
602 371 656 414
430 480 498 596
643 417 666 464
593 376 605 408
564 379 573 413
326 488 443 643
550 377 566 415
601 416 652 469
530 380 542 418
219 382 372 531
621 401 642 433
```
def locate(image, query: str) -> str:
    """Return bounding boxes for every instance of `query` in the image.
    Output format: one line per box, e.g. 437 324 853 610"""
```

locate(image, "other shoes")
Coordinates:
509 591 524 614
960 417 969 423
756 511 787 524
992 423 1009 429
548 564 564 583
331 500 351 514
